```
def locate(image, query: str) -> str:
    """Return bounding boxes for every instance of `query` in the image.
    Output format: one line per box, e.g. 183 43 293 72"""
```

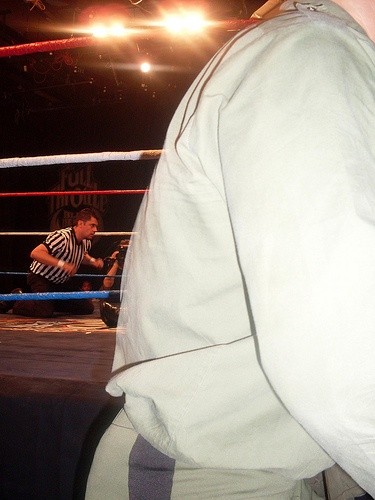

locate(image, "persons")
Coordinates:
80 280 99 315
0 208 103 318
101 239 130 303
84 0 375 500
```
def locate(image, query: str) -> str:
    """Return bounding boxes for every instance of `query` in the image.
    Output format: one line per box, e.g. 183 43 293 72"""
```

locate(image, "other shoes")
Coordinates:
0 288 23 313
100 301 119 327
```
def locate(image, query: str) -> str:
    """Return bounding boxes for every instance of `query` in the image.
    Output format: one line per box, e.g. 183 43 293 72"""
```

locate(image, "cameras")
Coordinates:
118 248 126 260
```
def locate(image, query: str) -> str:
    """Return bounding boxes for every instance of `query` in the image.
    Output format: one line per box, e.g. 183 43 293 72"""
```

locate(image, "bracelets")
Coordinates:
57 259 64 269
89 258 97 266
